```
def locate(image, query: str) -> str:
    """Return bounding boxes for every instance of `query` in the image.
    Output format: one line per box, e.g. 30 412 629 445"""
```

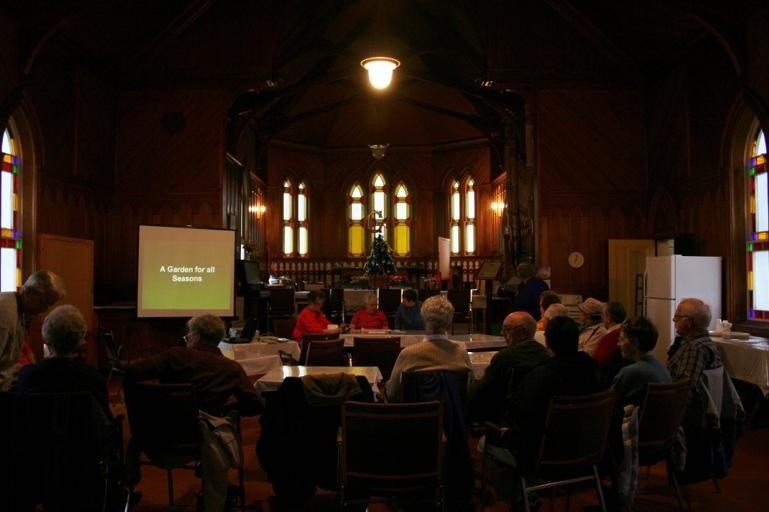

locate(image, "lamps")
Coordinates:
358 42 406 93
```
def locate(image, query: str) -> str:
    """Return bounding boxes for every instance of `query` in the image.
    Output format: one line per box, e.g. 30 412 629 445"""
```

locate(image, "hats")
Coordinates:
577 297 605 317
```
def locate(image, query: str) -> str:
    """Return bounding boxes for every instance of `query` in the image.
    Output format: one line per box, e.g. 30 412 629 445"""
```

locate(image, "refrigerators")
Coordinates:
642 256 723 370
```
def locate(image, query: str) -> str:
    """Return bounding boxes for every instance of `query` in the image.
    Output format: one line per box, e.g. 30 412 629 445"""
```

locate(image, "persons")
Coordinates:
349 294 391 335
575 297 608 354
129 314 267 430
593 302 625 356
665 298 717 406
516 262 547 317
1 266 66 392
538 293 564 316
385 295 474 398
611 316 674 403
291 290 342 342
13 304 116 447
470 311 553 444
541 317 612 397
543 302 568 322
393 290 428 330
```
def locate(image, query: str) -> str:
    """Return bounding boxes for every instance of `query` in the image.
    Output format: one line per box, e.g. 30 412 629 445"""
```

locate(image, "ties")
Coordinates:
667 336 683 361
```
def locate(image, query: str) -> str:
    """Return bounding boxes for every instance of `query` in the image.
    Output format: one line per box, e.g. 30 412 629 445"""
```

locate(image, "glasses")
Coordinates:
671 312 690 324
618 336 630 342
499 326 517 333
183 335 198 346
33 291 51 308
41 339 57 348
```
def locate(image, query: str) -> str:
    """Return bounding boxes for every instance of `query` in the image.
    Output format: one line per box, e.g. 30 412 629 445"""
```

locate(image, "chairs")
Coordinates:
627 372 697 501
446 286 479 329
505 396 613 511
264 286 299 332
117 374 249 508
338 395 454 512
303 337 347 367
317 286 348 326
352 335 403 379
267 373 375 465
374 286 405 316
